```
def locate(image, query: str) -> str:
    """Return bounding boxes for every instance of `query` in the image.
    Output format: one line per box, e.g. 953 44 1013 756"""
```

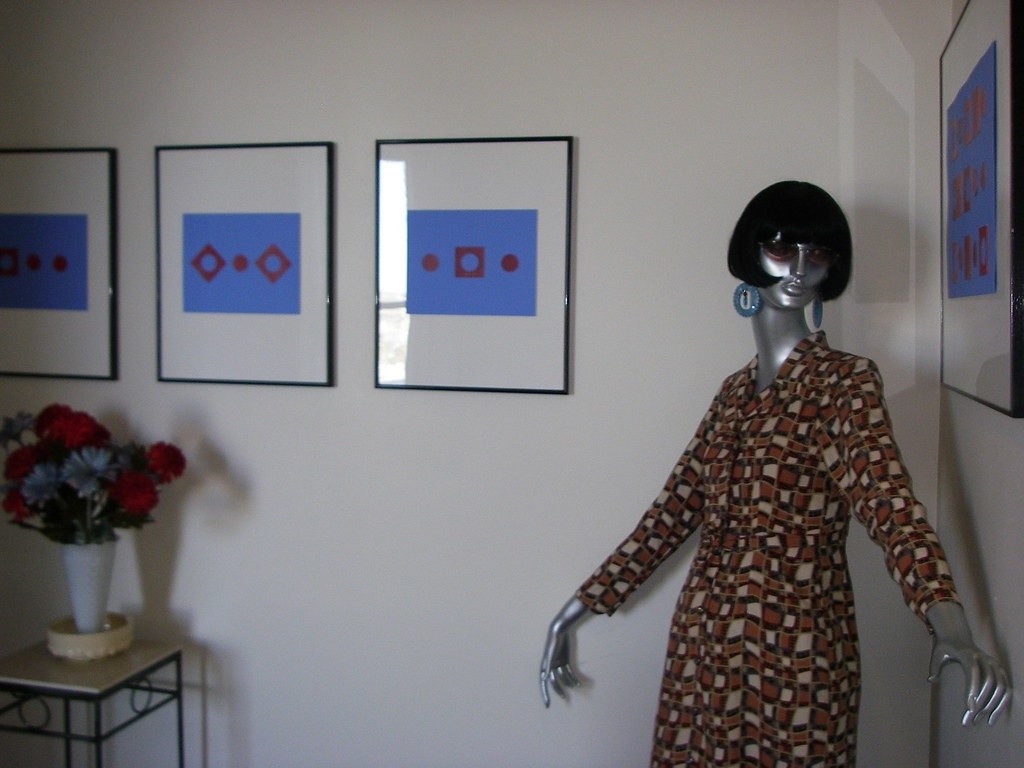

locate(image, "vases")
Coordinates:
60 542 115 633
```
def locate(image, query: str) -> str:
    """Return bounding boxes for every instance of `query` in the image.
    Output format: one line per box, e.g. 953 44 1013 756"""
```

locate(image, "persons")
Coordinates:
540 181 1017 768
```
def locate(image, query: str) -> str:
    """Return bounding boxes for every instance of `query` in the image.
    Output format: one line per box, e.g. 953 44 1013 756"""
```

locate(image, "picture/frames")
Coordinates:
152 140 335 389
939 0 1024 418
375 135 569 396
0 147 122 383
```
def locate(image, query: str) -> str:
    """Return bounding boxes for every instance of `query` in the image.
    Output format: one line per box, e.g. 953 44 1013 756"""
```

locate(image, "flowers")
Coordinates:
0 402 187 545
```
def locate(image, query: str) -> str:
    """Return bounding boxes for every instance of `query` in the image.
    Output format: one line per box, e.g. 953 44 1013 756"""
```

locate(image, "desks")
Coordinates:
0 639 185 768
